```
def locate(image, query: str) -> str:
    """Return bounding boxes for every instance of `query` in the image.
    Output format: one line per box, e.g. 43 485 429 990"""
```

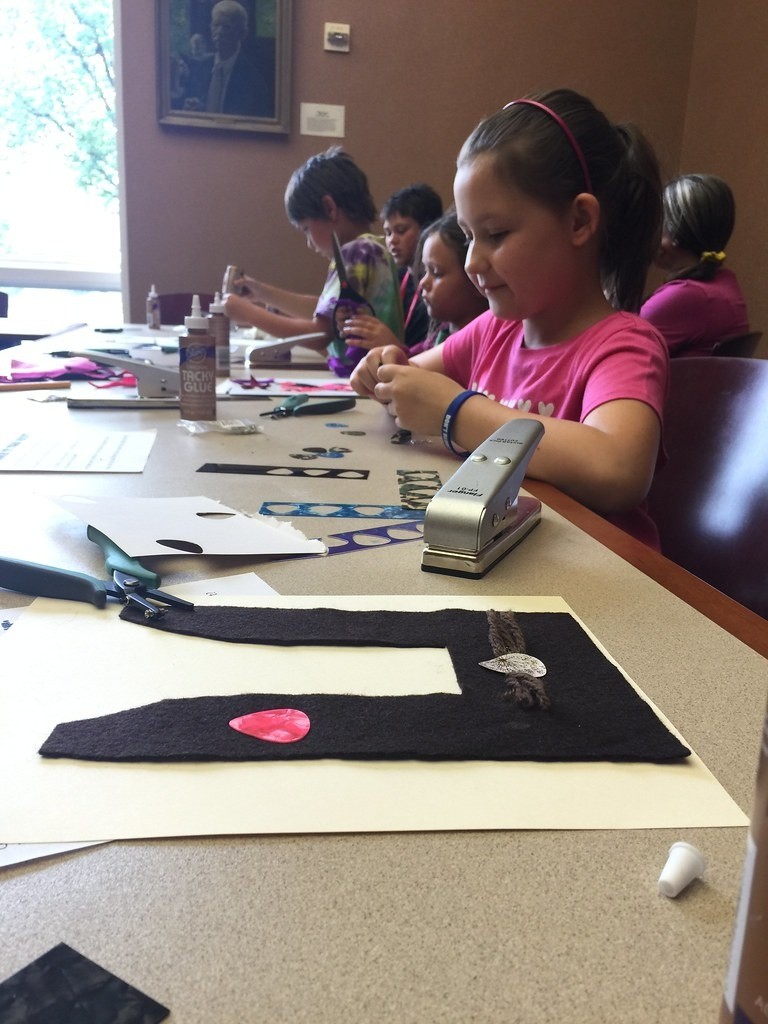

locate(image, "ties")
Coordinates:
206 63 223 114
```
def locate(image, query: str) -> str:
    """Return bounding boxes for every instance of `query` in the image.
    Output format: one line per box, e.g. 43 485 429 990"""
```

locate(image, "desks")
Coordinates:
0 324 768 1024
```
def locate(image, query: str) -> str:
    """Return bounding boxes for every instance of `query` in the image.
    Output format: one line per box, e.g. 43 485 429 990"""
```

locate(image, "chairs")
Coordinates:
645 327 768 621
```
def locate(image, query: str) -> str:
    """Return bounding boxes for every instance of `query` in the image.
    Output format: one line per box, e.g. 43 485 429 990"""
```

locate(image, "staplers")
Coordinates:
422 418 545 580
244 331 331 370
67 350 181 409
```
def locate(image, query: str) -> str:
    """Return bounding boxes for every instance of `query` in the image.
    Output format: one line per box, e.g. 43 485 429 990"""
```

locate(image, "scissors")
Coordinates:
331 228 377 342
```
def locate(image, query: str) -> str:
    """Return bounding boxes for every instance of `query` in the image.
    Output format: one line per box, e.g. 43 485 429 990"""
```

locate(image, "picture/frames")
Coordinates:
155 0 293 134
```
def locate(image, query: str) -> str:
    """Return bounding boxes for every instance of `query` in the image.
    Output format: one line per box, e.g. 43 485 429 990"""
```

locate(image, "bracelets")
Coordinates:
441 391 484 460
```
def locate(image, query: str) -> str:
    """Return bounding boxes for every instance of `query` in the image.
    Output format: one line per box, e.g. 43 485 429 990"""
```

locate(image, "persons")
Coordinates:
168 0 271 120
349 89 662 554
207 144 750 374
